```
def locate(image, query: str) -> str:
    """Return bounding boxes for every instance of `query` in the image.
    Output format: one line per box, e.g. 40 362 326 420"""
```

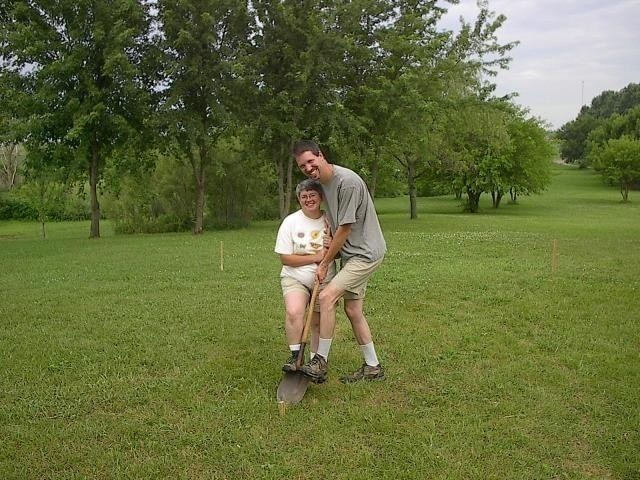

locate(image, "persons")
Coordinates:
274 179 336 383
293 140 386 384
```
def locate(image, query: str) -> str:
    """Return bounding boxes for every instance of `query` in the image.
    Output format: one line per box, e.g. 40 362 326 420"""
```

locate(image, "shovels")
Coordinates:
277 217 332 403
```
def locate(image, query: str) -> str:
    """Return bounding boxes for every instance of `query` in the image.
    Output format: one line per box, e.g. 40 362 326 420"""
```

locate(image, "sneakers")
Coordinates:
282 350 329 384
339 364 387 385
